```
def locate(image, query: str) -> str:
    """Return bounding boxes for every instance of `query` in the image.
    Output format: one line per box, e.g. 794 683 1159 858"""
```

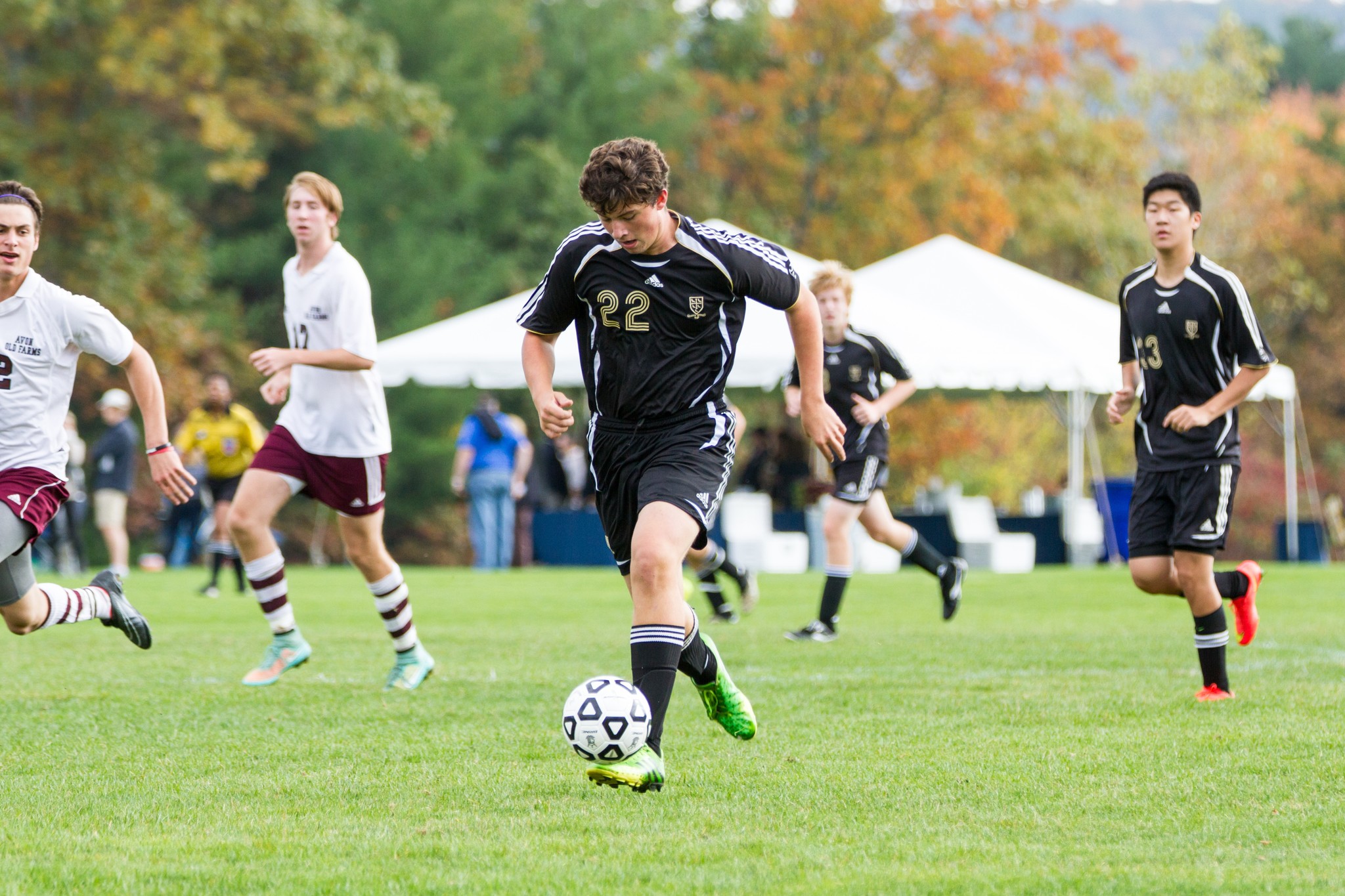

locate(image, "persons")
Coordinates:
44 411 94 574
178 366 254 596
88 388 140 580
225 169 437 690
741 423 776 492
780 256 964 641
516 136 846 796
488 399 531 499
684 380 760 621
449 393 516 568
537 434 588 511
768 426 808 513
1106 174 1261 700
157 418 203 568
1 178 197 652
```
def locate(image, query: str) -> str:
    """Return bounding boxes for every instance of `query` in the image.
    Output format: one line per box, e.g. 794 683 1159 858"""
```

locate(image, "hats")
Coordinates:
97 387 132 412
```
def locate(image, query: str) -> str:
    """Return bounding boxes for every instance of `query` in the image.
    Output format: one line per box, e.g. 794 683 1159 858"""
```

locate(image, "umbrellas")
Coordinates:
377 220 849 389
729 229 1301 562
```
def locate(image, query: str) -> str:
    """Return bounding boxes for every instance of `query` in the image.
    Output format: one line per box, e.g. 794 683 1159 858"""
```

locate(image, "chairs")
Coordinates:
715 485 1117 581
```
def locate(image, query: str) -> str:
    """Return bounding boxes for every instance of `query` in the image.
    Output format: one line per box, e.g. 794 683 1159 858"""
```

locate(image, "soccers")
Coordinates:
562 675 653 768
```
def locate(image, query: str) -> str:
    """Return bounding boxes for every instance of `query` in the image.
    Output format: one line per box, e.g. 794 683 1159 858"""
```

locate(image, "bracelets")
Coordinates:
145 442 174 456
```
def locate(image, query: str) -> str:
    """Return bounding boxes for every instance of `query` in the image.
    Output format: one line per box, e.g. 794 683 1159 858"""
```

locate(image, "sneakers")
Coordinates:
690 632 757 740
708 610 740 626
90 570 152 650
234 588 251 595
587 742 665 793
784 619 839 643
201 583 219 594
1228 559 1262 647
940 556 969 621
384 649 435 690
741 563 758 611
1194 684 1234 700
244 630 311 685
